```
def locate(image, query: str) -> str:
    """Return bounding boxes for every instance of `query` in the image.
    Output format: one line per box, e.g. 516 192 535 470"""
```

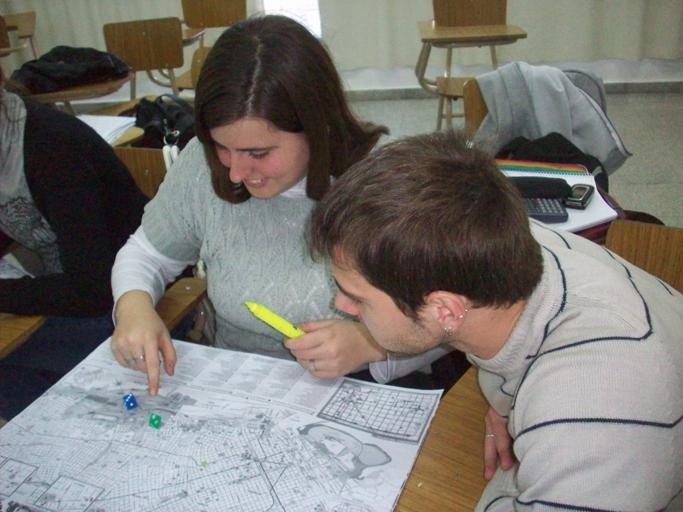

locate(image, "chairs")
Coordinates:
0 0 249 200
605 219 682 291
416 1 527 147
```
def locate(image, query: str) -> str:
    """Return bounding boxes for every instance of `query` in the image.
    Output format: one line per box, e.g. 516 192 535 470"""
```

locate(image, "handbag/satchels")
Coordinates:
116 94 198 154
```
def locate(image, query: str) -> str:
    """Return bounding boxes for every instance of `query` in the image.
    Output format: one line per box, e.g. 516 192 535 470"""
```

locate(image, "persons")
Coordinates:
0 69 195 423
305 132 683 512
110 14 460 396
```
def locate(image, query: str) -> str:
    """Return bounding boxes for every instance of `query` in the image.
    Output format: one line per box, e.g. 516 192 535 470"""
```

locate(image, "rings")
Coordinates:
127 359 136 365
134 355 143 362
308 359 316 372
486 433 495 437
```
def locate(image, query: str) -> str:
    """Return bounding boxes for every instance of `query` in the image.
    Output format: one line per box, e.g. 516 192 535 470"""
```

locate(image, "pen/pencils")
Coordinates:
245 302 304 339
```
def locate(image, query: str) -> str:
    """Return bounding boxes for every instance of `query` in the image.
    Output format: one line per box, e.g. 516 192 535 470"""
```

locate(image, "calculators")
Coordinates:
522 197 568 223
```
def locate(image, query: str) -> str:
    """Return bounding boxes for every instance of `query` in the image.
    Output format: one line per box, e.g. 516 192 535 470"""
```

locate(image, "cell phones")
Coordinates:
566 184 594 208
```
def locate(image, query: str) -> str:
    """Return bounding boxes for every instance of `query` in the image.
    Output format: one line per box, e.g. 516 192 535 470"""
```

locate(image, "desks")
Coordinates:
0 278 423 512
0 313 47 359
393 363 502 511
492 156 627 245
